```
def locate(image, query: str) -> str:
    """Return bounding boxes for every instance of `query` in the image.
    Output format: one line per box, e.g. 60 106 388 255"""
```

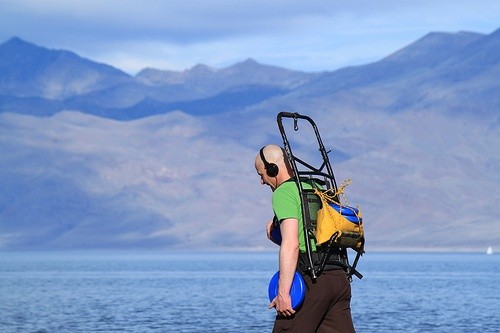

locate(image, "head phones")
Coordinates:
260 146 279 177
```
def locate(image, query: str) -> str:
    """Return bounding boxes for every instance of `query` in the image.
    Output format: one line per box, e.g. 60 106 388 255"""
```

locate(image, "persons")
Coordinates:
255 144 356 333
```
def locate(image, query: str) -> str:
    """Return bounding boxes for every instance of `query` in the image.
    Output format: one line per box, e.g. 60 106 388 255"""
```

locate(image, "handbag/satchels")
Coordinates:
313 187 362 247
